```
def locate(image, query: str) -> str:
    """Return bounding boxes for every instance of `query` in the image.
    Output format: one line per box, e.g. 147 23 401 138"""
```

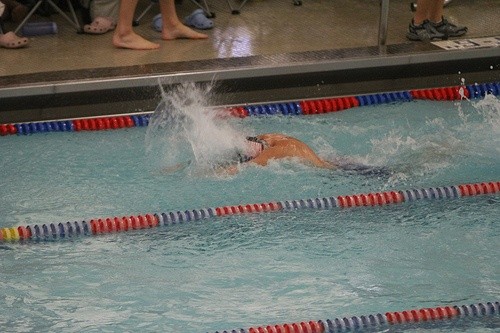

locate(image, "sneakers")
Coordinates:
421 17 468 37
408 20 447 41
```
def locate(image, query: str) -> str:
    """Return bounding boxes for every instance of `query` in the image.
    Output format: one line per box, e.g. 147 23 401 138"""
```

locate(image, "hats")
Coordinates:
237 136 264 161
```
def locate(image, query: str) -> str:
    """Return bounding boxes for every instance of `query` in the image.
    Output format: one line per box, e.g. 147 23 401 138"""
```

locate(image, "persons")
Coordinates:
407 0 468 42
112 0 209 50
150 132 396 182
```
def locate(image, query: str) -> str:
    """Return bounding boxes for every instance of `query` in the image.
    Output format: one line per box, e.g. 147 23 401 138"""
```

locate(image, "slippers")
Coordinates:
183 9 213 29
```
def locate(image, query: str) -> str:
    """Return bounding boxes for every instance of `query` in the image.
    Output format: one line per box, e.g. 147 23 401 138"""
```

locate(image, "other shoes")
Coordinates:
84 16 115 35
153 13 164 32
0 31 29 49
